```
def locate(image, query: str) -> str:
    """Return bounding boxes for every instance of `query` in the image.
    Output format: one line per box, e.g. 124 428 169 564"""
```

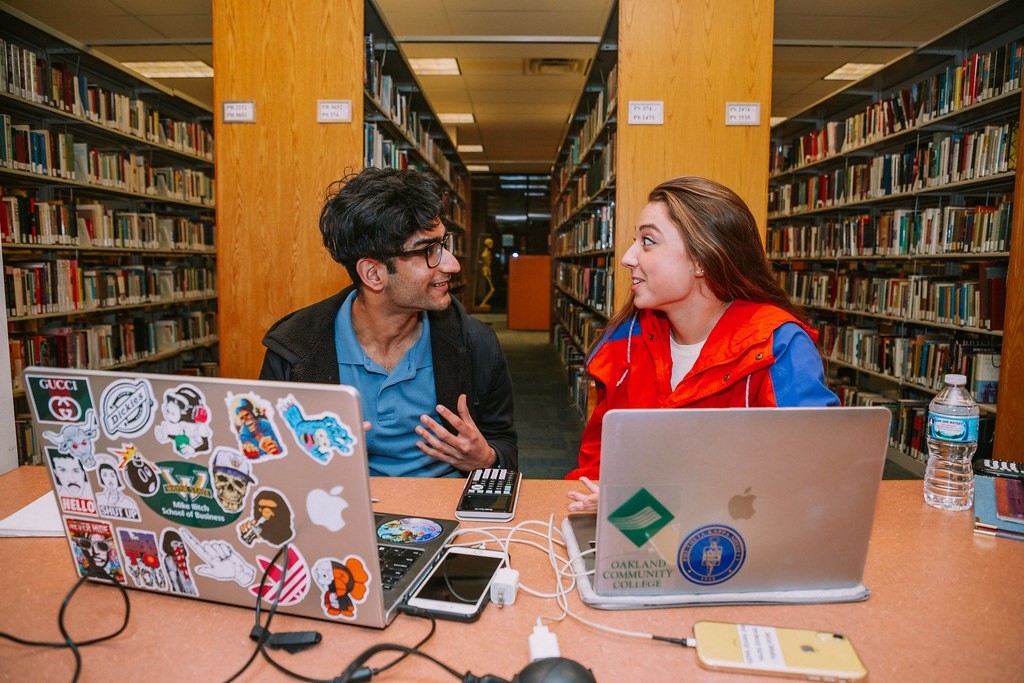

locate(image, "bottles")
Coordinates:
923 374 980 512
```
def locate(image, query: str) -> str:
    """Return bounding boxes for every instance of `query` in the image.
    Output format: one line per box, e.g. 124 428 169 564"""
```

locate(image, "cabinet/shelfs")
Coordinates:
211 0 474 381
546 0 772 424
0 0 214 477
765 0 1024 488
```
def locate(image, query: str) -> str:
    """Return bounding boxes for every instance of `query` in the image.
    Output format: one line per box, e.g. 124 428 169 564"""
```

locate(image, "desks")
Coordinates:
0 465 1024 683
506 254 554 331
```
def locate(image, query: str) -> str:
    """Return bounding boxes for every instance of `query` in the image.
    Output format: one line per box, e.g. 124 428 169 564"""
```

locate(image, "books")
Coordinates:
0 488 65 538
0 38 219 467
551 62 619 427
972 473 1024 540
362 33 467 309
766 38 1024 467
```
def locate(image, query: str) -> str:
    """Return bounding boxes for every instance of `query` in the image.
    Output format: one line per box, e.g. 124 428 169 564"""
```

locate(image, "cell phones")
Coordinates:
404 546 510 625
691 621 868 683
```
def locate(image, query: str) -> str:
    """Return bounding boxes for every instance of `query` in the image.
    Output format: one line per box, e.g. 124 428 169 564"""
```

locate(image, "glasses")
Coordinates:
377 232 454 268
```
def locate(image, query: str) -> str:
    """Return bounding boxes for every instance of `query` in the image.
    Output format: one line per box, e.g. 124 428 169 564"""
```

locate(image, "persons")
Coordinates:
480 238 496 308
257 166 518 478
562 175 843 513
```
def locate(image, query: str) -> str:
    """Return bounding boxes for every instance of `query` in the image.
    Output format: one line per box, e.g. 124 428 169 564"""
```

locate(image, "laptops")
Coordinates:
563 407 894 612
20 366 462 629
